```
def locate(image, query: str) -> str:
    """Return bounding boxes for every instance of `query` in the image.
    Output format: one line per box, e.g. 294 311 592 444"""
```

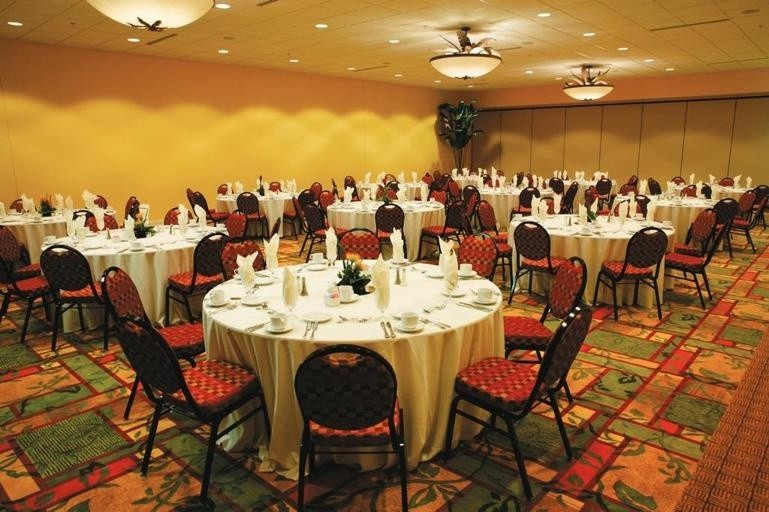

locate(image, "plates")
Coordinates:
331 201 441 212
44 224 225 251
511 213 673 237
204 257 496 334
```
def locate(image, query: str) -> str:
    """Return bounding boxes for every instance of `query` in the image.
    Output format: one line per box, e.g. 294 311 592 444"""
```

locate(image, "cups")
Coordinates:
209 253 493 330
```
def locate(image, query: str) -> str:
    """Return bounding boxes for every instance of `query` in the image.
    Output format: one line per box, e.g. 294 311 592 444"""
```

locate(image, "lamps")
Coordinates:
429 27 504 80
85 0 216 32
563 65 613 102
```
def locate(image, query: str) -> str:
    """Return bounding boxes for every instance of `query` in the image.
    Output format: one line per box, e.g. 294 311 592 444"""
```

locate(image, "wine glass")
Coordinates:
0 191 97 218
343 184 429 210
66 217 206 248
532 195 656 227
